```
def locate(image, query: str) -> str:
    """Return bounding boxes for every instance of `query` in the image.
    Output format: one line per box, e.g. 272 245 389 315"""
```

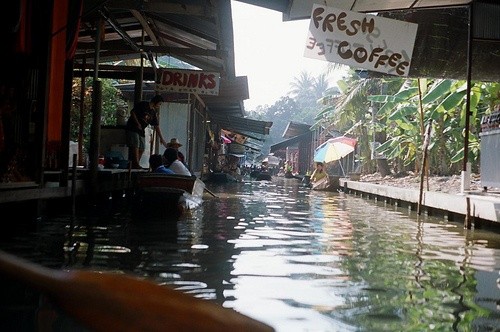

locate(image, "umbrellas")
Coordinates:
313 136 356 180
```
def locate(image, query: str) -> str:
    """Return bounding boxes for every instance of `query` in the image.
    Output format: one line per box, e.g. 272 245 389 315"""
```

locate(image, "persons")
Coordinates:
310 162 327 182
124 95 164 169
149 138 192 176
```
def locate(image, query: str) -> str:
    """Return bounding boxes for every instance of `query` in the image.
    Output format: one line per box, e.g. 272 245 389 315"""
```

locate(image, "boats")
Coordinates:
250 169 271 180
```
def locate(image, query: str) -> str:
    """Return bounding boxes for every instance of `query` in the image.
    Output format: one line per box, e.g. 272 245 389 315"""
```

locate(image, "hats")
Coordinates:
166 138 184 147
